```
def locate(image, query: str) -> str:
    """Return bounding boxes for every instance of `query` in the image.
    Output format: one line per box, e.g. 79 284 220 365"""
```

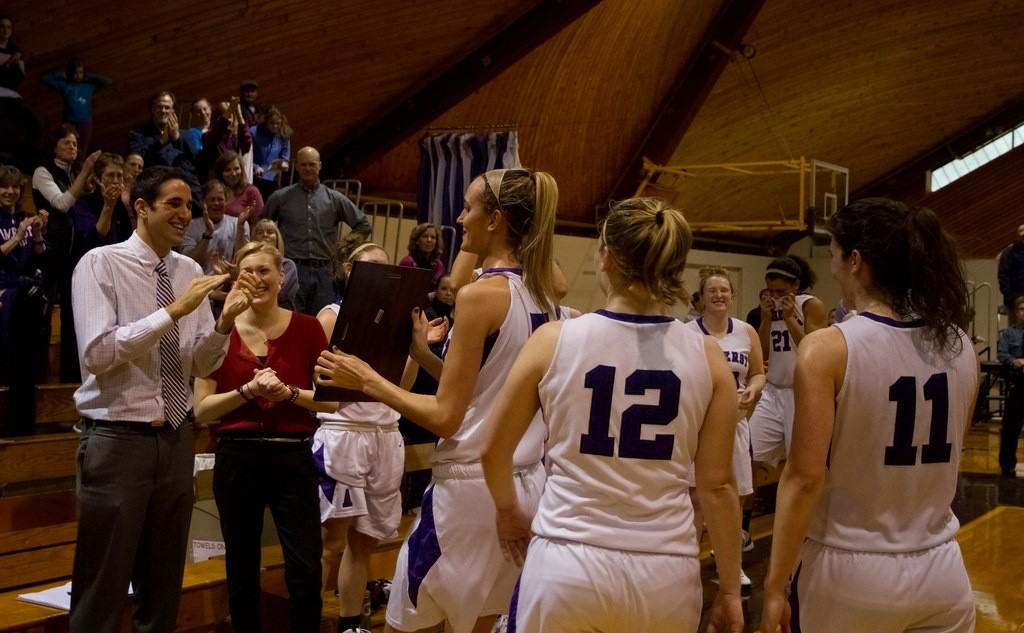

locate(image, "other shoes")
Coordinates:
343 628 371 633
1002 469 1016 478
740 567 751 588
710 538 755 556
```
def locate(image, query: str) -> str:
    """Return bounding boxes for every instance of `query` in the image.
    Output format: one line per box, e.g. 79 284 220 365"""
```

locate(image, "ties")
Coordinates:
154 259 188 430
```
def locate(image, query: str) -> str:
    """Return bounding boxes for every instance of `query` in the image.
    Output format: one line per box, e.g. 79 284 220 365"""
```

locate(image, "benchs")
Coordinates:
0 170 417 633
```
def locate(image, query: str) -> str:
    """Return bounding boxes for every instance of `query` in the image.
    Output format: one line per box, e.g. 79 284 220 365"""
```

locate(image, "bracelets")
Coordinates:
287 384 299 401
247 383 256 397
237 386 250 402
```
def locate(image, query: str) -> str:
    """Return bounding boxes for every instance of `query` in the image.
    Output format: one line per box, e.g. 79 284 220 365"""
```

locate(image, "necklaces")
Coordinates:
249 322 279 345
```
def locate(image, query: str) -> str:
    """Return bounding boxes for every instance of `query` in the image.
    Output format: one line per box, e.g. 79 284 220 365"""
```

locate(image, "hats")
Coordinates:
241 79 258 89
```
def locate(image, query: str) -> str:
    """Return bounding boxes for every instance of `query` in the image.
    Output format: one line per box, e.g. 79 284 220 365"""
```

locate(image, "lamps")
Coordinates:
738 43 757 59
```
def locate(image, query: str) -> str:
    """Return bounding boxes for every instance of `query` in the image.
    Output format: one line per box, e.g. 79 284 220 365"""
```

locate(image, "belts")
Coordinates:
214 436 311 442
86 415 189 427
293 259 333 266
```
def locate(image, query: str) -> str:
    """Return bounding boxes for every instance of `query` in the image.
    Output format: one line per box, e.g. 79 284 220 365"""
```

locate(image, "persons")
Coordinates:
399 272 457 360
398 223 446 302
69 164 260 633
684 267 766 585
746 288 771 332
996 296 1024 478
480 196 745 633
0 15 38 165
312 233 449 633
998 224 1024 325
40 59 112 158
759 196 982 633
313 167 584 633
710 255 828 558
193 241 340 633
0 79 373 438
